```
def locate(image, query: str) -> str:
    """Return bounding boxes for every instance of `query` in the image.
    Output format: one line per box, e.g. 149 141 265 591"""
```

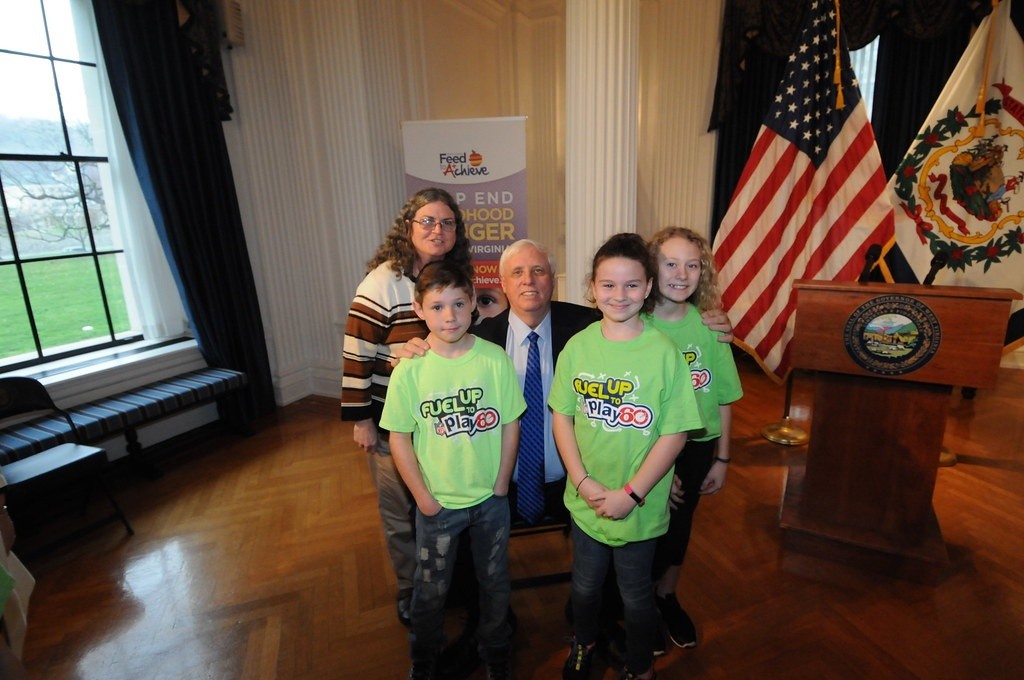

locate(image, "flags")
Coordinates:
709 0 898 391
883 2 1024 363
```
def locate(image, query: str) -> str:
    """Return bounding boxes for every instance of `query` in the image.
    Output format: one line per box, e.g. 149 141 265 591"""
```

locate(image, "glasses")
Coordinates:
413 217 457 232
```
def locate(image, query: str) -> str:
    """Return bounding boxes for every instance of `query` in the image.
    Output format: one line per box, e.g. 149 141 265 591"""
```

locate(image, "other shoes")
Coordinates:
653 584 696 648
408 659 433 680
600 620 628 669
653 629 666 656
486 659 512 680
561 633 596 680
397 587 416 626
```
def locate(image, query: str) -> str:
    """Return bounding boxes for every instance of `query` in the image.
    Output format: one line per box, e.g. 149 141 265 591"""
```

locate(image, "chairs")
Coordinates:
0 377 134 566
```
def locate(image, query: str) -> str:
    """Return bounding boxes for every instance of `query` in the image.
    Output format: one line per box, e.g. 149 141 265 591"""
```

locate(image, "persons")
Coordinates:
474 287 508 326
377 260 530 680
341 186 472 635
545 233 703 680
386 239 734 680
636 227 743 661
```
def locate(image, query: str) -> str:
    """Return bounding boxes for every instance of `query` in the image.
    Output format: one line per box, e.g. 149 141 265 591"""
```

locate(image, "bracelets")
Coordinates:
624 483 646 508
576 474 590 498
714 456 732 463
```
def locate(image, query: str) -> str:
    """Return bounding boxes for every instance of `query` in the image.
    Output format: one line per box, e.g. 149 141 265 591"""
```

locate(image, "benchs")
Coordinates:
0 367 252 491
